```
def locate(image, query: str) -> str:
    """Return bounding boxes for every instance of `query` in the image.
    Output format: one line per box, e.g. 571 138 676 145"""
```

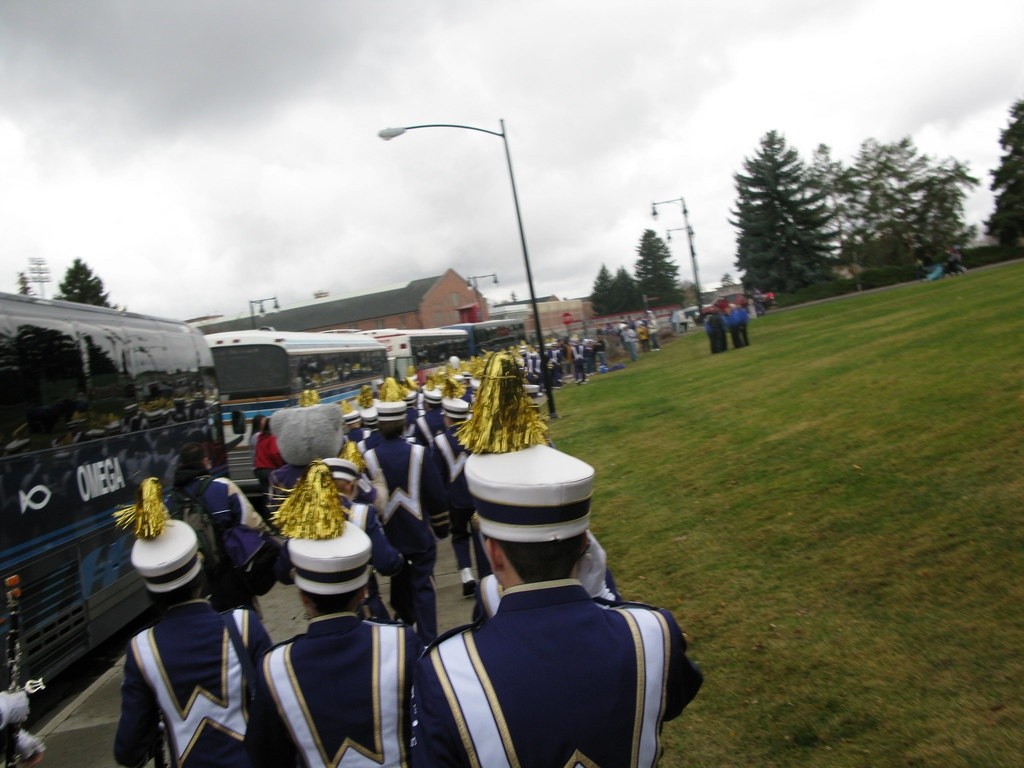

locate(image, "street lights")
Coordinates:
376 117 558 421
250 297 280 330
466 273 500 321
651 196 705 328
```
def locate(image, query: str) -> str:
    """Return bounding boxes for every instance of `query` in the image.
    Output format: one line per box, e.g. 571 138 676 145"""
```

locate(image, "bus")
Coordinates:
204 326 391 498
361 328 470 387
0 290 228 697
441 316 526 358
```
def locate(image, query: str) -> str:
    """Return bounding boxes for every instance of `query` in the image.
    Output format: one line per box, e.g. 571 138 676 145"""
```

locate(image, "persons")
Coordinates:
246 514 430 768
0 689 46 768
1 386 222 534
943 246 967 278
377 310 662 393
163 442 272 611
249 389 622 652
412 448 703 768
111 519 279 768
292 361 373 389
704 304 748 353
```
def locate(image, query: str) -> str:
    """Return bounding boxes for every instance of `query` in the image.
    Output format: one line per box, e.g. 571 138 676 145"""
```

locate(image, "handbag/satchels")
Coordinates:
222 525 281 595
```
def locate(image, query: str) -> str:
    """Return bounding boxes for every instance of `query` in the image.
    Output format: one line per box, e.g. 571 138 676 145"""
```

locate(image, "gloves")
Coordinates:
16 728 46 758
0 691 29 729
576 530 615 610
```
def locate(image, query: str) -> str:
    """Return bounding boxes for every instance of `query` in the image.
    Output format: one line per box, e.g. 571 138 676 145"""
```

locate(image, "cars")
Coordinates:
671 305 710 330
694 292 775 323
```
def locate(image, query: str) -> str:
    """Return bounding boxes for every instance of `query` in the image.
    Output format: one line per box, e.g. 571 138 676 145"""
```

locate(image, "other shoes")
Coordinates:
463 580 479 595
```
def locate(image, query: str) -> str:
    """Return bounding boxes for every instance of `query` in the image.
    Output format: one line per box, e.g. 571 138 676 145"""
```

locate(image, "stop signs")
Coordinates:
563 313 573 326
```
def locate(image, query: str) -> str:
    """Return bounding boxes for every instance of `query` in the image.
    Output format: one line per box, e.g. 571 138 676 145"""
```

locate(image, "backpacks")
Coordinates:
171 476 223 575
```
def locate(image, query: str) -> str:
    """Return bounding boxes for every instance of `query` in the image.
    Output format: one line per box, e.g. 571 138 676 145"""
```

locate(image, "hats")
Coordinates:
321 458 372 493
287 520 373 595
342 342 559 425
465 445 595 543
5 394 219 456
131 520 204 593
